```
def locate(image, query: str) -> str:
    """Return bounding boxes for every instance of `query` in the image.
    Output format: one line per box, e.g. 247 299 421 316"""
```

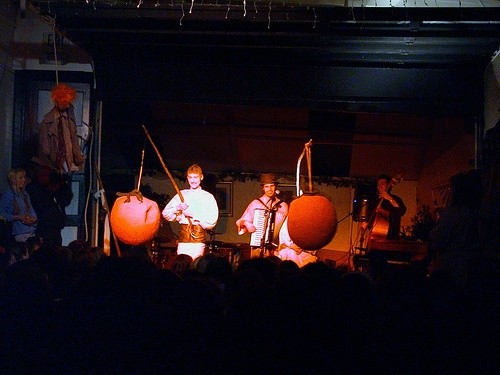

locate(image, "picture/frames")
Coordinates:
216 182 233 217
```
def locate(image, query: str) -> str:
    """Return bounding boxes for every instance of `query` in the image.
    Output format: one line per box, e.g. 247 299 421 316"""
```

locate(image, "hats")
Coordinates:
259 173 278 187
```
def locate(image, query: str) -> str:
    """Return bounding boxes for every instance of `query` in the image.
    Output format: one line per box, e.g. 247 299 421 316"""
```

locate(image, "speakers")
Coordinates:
353 180 380 222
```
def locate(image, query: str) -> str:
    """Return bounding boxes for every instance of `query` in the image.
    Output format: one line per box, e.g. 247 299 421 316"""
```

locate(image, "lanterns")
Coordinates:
110 194 161 246
286 189 338 251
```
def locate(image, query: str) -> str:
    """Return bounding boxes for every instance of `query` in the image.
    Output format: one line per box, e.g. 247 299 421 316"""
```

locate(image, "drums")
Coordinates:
218 247 235 264
204 244 217 257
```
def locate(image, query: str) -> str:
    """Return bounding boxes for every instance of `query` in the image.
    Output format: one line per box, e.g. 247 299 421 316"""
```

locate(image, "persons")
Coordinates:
25 166 65 246
0 167 38 244
38 85 85 205
1 236 500 375
235 172 289 257
361 173 406 241
161 163 219 257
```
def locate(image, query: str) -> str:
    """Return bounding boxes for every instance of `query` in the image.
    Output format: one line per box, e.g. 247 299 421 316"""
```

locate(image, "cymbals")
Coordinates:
151 236 170 243
206 230 222 235
232 243 250 249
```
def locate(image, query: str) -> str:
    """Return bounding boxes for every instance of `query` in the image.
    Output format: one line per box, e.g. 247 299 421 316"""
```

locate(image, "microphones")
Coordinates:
267 190 280 203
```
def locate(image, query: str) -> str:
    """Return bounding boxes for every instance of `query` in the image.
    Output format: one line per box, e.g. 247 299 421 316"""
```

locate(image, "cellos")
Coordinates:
366 175 405 246
